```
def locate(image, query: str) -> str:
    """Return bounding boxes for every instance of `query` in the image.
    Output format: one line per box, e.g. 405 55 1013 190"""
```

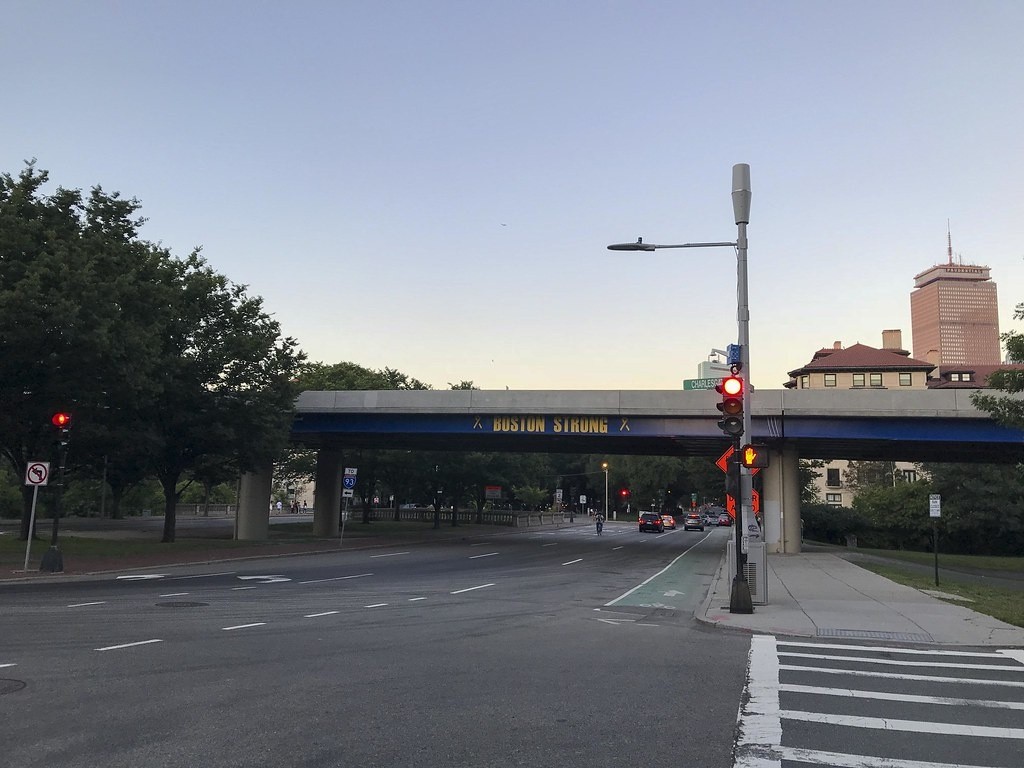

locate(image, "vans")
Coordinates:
402 503 423 510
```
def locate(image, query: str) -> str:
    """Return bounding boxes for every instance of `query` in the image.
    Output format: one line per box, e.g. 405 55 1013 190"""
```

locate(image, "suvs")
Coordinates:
683 513 705 532
638 511 666 533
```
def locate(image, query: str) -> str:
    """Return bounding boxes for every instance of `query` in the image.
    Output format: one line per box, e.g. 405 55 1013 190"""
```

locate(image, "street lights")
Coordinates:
601 461 609 522
607 236 753 614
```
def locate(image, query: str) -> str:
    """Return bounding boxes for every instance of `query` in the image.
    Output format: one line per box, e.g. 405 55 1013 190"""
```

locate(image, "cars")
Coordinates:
661 514 677 530
700 511 734 527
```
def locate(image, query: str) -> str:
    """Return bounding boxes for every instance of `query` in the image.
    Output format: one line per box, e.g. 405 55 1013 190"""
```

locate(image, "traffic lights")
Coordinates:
621 489 627 496
741 443 770 469
52 410 75 454
716 384 726 430
722 376 745 436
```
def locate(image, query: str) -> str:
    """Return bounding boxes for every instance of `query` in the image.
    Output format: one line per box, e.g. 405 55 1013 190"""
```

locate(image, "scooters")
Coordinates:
595 518 603 536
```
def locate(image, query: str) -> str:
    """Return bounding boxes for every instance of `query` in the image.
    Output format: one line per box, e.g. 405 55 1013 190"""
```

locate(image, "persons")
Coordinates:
269 500 273 514
303 500 307 513
290 501 294 513
801 518 805 543
756 514 762 530
276 500 283 515
595 511 604 533
